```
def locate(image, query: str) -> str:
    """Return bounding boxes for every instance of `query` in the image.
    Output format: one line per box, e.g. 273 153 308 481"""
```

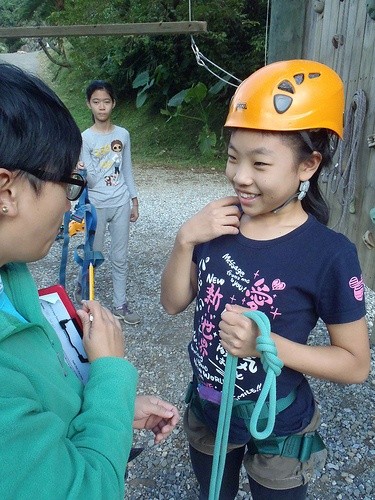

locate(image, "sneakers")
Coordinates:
73 279 84 305
113 304 141 324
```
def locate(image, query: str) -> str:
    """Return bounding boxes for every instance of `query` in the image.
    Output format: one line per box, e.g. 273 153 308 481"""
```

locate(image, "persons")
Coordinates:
155 57 373 500
72 80 142 326
0 59 185 500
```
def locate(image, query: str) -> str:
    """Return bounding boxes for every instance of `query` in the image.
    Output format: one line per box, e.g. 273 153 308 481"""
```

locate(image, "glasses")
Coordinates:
8 165 87 201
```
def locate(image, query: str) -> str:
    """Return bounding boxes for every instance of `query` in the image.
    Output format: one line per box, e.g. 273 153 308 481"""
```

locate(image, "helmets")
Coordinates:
223 59 346 140
69 218 86 237
